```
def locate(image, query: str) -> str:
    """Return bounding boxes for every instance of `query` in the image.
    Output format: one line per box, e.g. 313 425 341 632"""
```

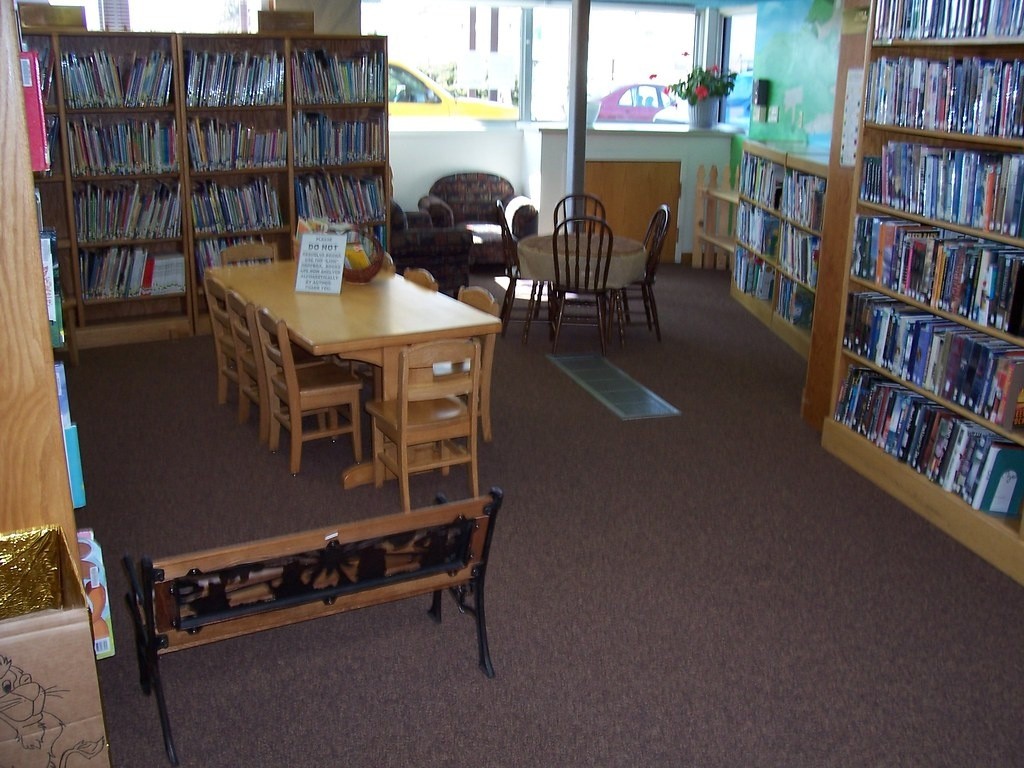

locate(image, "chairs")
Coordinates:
341 267 439 402
256 306 364 472
607 202 672 346
364 338 481 509
204 274 228 424
228 288 327 452
553 191 606 234
496 200 560 341
546 215 613 356
459 283 500 444
220 242 279 266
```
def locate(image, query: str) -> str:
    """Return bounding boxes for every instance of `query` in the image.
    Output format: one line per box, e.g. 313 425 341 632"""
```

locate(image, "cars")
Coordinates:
388 59 531 119
588 82 691 125
653 72 787 129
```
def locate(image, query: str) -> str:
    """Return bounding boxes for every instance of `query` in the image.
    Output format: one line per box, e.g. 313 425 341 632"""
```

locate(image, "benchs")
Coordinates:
123 486 505 768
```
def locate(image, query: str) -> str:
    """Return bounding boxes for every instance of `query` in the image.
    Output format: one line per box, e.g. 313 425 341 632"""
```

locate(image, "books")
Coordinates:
834 0 1024 513
61 46 386 302
19 43 116 661
736 151 827 332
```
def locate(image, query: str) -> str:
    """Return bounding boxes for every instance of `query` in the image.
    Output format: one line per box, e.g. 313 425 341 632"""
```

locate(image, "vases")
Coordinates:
686 99 721 128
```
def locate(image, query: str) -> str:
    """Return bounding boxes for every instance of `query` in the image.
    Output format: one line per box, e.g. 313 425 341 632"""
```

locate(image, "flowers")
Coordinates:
652 51 739 105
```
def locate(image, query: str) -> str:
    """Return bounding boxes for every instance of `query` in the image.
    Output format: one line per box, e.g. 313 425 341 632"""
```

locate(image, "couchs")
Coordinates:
418 172 538 270
388 165 472 300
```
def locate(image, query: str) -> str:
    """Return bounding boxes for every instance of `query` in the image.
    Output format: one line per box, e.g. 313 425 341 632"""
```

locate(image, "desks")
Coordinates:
205 262 501 489
517 234 647 342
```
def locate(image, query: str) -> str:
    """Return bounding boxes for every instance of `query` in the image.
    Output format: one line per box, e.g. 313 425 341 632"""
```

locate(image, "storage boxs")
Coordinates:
76 531 116 661
257 9 314 35
18 1 88 31
0 524 115 768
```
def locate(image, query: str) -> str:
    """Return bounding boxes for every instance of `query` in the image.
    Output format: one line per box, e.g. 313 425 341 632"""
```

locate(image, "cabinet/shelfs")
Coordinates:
0 0 389 663
801 0 1024 588
728 140 832 364
